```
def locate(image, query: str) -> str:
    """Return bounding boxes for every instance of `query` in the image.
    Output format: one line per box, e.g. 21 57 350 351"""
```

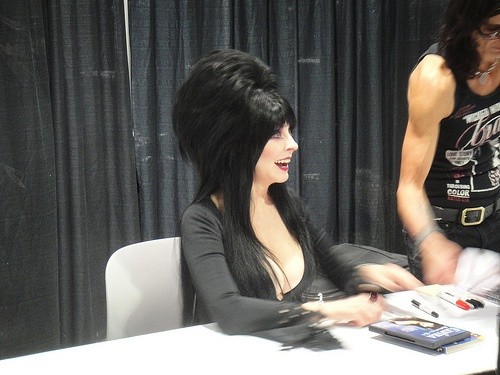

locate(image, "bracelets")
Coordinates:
409 226 443 247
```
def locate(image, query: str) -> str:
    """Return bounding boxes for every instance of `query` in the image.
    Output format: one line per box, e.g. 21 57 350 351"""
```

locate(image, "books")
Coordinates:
369 315 485 356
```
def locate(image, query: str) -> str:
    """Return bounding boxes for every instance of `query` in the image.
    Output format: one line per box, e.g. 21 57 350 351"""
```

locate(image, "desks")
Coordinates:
0 284 500 375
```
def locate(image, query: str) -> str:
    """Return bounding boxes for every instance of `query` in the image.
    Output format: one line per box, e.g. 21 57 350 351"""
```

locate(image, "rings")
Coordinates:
368 290 378 302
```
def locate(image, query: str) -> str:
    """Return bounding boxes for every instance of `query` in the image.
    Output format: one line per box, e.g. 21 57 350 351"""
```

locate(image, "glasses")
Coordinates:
477 26 500 40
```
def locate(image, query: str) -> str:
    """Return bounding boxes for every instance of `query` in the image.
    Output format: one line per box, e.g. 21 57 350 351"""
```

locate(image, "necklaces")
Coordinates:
475 57 500 86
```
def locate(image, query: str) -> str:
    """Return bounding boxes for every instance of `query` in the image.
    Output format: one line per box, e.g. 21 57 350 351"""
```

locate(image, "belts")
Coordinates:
431 198 499 225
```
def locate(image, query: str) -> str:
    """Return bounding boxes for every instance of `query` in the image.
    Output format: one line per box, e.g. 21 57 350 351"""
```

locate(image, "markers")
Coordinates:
370 292 377 302
412 299 439 318
439 290 485 310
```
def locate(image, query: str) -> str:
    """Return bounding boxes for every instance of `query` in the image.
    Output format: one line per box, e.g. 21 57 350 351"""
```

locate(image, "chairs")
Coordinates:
105 237 183 340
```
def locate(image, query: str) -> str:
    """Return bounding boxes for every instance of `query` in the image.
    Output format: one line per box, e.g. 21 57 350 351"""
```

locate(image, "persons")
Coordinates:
396 0 500 284
171 48 424 336
